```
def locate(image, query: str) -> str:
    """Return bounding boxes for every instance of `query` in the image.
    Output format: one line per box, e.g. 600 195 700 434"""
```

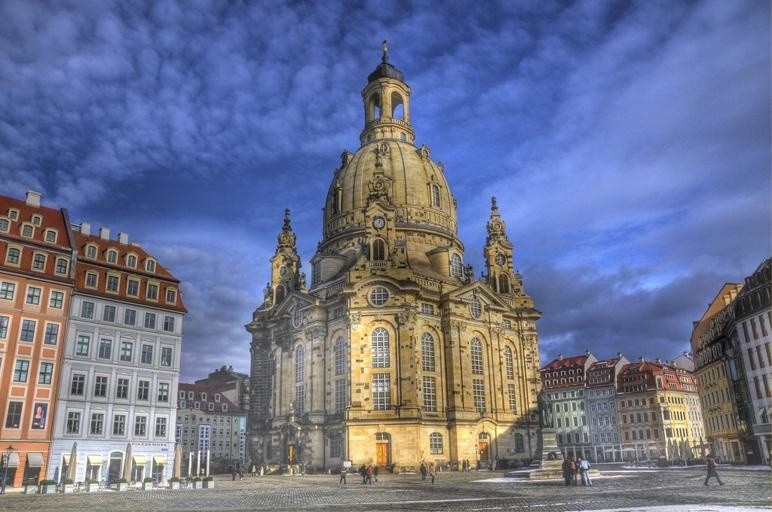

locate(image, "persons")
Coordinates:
231 466 237 480
491 461 497 472
365 464 375 484
462 459 467 472
561 455 593 487
238 466 244 480
33 404 46 428
429 462 437 485
466 458 471 472
339 465 347 485
251 463 257 476
702 454 725 486
257 463 261 475
420 461 428 480
260 463 264 476
358 462 367 484
